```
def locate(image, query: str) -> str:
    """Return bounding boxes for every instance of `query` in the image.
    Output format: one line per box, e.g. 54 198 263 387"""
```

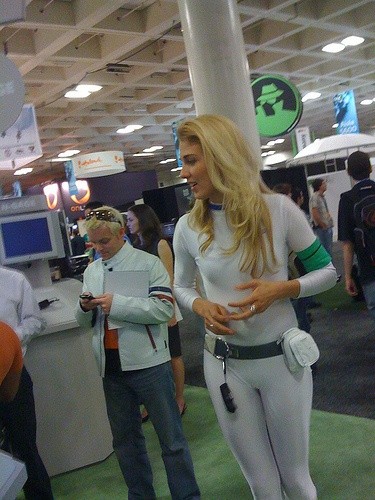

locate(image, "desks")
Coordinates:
23 278 115 480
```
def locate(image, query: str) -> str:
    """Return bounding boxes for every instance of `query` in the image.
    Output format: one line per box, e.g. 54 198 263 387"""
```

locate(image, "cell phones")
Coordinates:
80 294 95 300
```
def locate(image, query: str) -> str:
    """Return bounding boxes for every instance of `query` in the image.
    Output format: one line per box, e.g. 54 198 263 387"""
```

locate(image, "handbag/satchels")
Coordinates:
277 326 321 375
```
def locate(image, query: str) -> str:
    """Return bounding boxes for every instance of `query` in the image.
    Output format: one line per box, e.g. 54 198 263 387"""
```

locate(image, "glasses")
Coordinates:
84 210 124 229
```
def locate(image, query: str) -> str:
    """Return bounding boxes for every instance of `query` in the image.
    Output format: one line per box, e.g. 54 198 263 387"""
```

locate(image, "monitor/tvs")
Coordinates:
0 212 58 265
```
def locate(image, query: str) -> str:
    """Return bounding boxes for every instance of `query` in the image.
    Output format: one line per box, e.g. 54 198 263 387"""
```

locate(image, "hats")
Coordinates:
346 150 371 177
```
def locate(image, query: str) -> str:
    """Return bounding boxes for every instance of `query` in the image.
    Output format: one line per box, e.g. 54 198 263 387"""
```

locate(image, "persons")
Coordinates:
170 114 336 499
307 176 341 282
125 205 188 424
271 183 311 336
74 207 201 499
1 267 54 499
337 151 375 324
289 187 323 322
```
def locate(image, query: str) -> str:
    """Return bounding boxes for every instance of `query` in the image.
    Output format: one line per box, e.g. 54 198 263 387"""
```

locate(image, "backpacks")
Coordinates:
339 189 375 257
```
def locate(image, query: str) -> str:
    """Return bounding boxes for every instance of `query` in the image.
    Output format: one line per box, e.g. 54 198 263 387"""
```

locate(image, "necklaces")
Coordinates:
206 201 226 211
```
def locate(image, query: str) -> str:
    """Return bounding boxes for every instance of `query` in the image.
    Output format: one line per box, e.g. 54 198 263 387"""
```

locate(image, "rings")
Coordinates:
250 304 257 313
208 324 212 329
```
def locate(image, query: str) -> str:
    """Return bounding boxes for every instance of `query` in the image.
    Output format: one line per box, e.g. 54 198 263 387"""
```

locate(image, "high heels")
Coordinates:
176 402 188 418
139 410 150 424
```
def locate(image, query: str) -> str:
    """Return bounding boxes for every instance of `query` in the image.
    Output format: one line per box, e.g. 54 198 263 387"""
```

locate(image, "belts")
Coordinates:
205 333 286 359
